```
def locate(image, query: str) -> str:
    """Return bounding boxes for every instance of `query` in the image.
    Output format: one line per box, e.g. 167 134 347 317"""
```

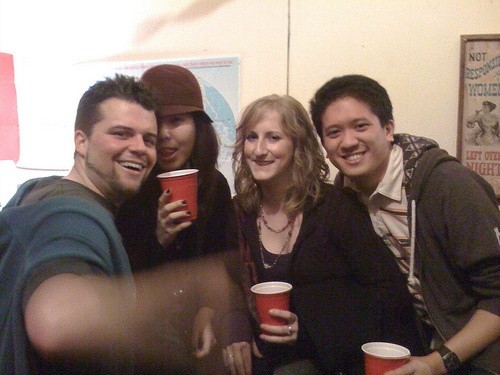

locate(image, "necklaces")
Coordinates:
255 195 296 269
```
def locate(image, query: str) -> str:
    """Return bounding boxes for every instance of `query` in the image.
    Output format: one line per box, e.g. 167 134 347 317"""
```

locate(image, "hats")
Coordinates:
138 64 204 116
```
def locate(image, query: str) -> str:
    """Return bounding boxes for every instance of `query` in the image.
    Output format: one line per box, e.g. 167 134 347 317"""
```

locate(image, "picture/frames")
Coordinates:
456 34 500 205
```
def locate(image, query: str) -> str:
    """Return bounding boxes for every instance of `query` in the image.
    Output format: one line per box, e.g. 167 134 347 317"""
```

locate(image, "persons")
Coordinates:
309 75 500 375
0 64 238 375
231 94 417 375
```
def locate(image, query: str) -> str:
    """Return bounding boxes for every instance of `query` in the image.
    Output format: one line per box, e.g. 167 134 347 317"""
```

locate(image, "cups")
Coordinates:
250 281 293 337
361 342 411 375
157 169 199 223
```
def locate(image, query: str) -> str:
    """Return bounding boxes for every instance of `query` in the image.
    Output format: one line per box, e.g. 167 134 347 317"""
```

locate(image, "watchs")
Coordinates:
436 344 462 375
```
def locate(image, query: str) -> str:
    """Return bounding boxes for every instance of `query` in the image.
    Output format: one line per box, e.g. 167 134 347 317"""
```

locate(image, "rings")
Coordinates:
225 354 232 359
288 325 292 335
